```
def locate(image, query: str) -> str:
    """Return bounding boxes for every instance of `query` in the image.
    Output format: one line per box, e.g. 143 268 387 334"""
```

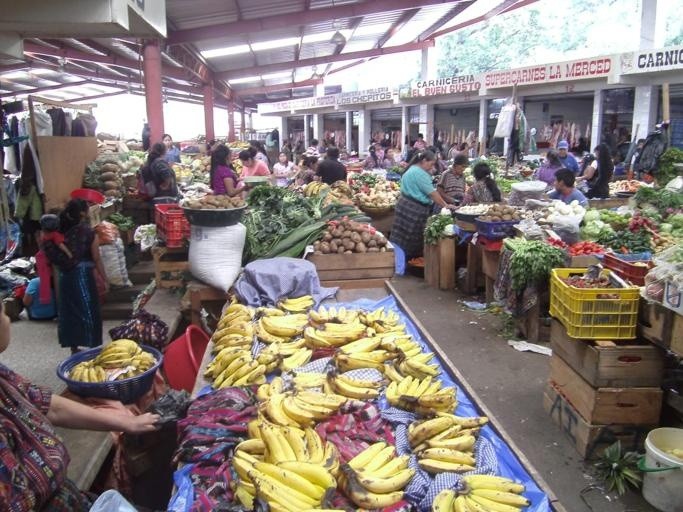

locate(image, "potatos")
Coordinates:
183 195 244 209
480 205 520 221
313 222 387 255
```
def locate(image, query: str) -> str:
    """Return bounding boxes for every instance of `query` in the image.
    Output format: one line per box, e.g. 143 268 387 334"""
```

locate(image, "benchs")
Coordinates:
45 289 189 496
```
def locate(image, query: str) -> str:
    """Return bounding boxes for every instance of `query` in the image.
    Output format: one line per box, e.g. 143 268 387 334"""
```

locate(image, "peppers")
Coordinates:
599 226 650 254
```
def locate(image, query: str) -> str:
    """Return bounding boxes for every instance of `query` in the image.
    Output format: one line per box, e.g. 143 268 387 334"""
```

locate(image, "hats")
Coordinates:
558 140 569 149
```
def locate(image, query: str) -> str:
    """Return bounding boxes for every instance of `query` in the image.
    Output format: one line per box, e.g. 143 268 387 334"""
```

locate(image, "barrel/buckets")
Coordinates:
638 427 683 512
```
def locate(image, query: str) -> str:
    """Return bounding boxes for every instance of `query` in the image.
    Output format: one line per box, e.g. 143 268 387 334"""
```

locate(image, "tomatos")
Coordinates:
569 242 603 256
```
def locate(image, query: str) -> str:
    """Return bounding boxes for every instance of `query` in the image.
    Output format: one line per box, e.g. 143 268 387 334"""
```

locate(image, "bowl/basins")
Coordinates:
179 200 248 227
69 189 103 203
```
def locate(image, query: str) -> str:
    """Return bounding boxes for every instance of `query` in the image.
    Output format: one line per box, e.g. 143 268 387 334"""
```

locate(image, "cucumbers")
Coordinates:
321 204 372 222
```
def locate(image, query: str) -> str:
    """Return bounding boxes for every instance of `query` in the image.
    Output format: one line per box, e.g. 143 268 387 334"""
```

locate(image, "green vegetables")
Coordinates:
502 237 565 291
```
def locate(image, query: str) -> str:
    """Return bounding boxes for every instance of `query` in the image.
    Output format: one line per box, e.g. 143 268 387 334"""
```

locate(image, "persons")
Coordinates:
206 130 346 197
161 135 180 162
535 127 647 209
37 214 79 271
0 296 160 511
142 141 180 280
363 134 503 280
22 263 57 320
56 199 105 354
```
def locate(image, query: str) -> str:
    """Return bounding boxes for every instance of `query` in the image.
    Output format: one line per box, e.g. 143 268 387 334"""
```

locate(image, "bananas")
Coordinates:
331 442 414 508
432 474 532 512
303 180 326 196
203 294 458 415
406 409 488 473
233 390 348 512
70 338 155 383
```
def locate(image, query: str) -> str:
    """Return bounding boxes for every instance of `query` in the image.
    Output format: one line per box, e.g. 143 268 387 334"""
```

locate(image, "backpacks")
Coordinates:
136 158 169 200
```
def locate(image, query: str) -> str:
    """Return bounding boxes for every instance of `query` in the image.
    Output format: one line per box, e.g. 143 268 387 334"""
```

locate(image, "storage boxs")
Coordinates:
545 266 668 467
148 202 190 289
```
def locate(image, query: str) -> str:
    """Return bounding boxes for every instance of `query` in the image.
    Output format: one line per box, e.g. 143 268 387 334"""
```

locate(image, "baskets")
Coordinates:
154 204 191 248
474 217 520 241
362 206 392 219
602 254 653 287
57 345 162 403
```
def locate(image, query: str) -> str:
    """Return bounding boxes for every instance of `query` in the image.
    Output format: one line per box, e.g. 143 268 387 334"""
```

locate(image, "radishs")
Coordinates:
101 163 119 196
